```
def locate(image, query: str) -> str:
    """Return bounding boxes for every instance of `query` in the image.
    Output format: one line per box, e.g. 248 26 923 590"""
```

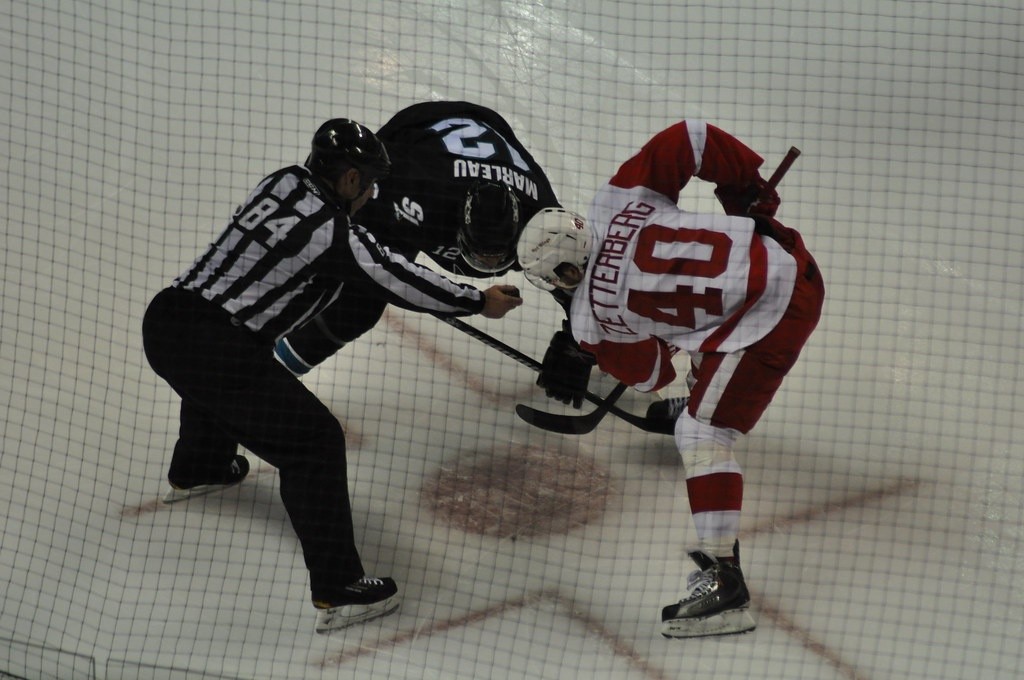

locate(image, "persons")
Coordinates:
516 117 827 623
140 116 524 609
266 100 597 411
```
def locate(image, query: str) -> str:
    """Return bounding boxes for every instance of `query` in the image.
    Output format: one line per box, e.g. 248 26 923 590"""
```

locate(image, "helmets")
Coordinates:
304 118 391 182
516 208 593 291
456 178 519 261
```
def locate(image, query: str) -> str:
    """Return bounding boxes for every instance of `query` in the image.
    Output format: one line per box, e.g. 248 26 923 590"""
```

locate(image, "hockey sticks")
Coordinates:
431 310 677 437
515 146 802 436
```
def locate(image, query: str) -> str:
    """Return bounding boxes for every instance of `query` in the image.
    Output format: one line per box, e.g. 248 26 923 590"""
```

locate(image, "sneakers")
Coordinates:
646 370 698 420
662 538 757 639
162 455 250 504
310 577 398 635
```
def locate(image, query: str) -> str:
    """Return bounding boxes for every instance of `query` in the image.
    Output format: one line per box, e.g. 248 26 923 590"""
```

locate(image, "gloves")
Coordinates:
714 178 782 216
535 319 598 410
